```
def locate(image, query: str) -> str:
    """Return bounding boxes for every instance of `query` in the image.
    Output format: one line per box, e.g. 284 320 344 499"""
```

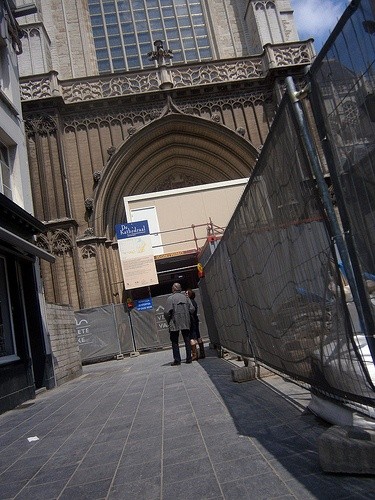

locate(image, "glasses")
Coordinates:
185 292 188 295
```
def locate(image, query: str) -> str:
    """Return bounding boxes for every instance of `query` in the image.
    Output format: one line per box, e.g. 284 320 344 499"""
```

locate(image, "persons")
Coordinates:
164 282 196 366
184 287 205 363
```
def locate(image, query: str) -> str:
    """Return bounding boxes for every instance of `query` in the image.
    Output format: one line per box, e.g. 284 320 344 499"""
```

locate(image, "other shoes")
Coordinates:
171 362 181 366
182 359 192 363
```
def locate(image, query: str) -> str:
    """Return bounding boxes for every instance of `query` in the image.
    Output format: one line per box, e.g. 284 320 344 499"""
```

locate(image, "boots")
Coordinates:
198 342 206 359
190 344 198 361
